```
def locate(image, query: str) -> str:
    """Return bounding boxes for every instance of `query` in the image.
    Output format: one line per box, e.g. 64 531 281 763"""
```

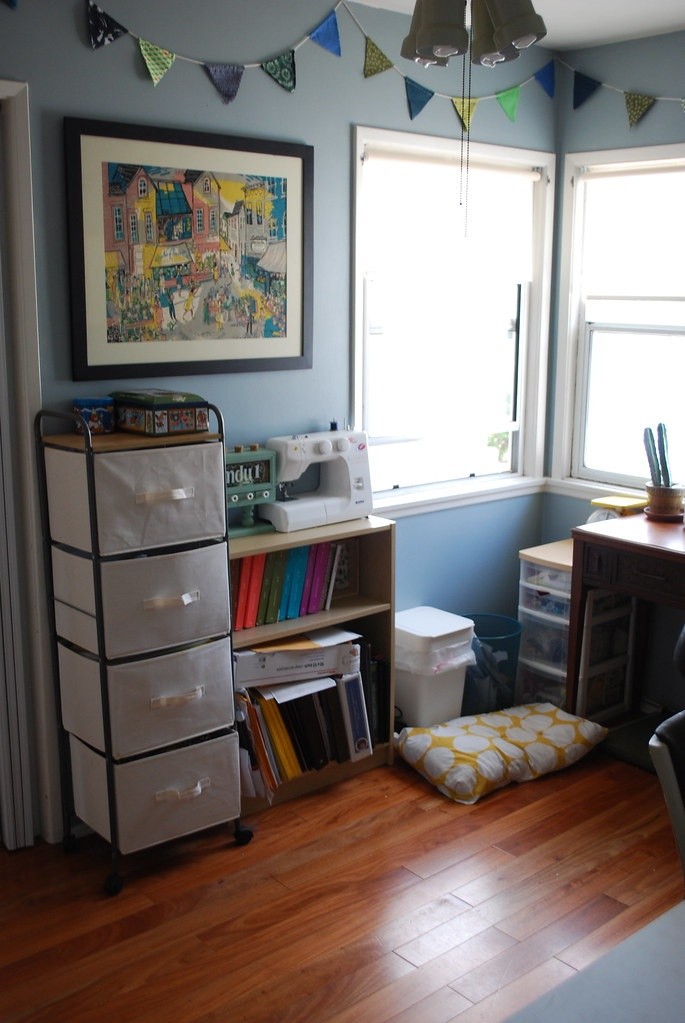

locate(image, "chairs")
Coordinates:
648 710 685 872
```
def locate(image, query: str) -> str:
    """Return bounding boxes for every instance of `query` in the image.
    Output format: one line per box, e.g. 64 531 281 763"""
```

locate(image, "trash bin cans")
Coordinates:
395 605 476 727
460 613 523 715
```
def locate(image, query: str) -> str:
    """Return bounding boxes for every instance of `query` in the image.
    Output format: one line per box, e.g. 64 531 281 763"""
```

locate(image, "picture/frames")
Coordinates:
63 116 313 382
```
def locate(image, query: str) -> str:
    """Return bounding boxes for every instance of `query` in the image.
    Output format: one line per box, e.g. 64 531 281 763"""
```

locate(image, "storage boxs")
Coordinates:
107 388 209 437
232 627 363 690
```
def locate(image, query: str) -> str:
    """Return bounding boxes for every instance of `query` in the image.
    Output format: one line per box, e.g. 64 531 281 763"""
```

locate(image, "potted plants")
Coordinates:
643 422 685 522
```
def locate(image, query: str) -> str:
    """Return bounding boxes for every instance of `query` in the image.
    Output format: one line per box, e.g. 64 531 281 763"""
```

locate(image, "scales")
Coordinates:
586 496 648 524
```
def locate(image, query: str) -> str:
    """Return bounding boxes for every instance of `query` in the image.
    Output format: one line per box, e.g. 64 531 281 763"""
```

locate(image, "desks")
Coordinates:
565 514 685 724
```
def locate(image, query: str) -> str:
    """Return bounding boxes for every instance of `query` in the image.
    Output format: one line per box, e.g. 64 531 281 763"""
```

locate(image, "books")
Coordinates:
230 542 342 631
233 641 381 800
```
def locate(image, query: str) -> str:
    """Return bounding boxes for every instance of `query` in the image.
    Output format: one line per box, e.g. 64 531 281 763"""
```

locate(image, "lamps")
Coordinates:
400 0 449 68
470 0 520 68
415 0 468 59
485 0 547 54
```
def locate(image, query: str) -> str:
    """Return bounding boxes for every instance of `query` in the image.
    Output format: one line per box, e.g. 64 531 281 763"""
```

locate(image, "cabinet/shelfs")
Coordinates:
518 538 575 710
33 401 256 897
227 515 397 819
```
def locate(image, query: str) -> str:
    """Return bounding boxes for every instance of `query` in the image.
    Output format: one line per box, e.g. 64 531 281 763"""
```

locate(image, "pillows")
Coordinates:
394 703 609 805
